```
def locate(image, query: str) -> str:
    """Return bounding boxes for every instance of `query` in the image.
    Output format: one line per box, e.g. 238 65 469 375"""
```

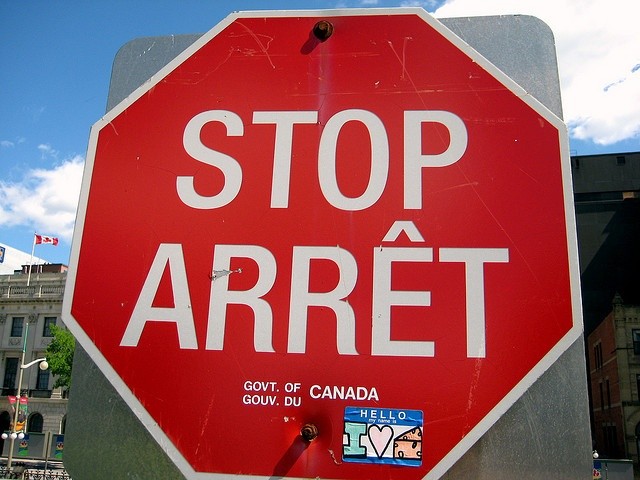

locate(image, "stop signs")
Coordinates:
60 7 585 480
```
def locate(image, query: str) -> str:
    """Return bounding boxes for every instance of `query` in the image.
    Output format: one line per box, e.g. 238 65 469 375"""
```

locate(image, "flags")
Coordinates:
35 233 60 246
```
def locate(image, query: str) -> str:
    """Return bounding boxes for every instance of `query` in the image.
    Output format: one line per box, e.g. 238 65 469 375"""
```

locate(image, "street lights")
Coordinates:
1 357 49 478
